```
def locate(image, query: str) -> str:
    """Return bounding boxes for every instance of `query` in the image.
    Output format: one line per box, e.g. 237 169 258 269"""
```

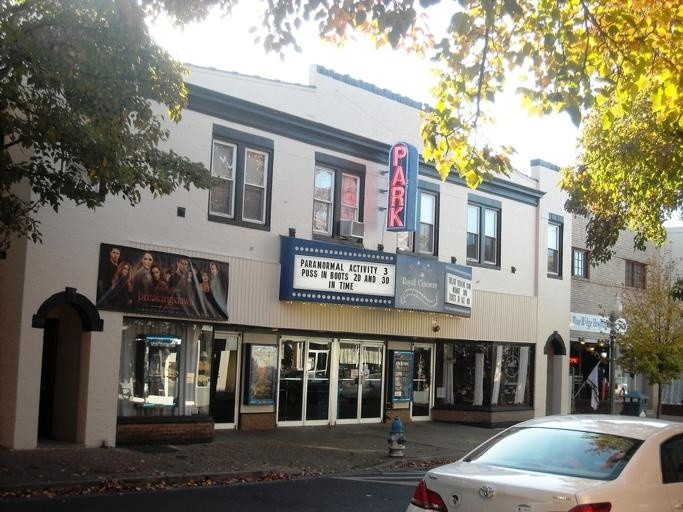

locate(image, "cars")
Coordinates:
279 370 381 408
402 413 682 512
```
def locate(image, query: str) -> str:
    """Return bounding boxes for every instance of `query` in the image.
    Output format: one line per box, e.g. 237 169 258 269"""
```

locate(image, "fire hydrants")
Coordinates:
385 415 406 460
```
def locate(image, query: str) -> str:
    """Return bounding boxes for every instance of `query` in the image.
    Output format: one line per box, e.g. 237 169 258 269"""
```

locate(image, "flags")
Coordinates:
584 359 599 411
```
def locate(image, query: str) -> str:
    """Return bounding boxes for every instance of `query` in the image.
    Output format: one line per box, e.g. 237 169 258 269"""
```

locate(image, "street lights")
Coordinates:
604 292 624 416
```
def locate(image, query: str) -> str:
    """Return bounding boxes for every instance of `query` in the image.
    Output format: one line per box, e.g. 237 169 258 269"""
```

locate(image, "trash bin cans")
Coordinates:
620 391 649 417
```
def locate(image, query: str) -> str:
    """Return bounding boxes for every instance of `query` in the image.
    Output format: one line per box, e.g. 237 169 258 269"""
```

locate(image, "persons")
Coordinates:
94 243 227 320
